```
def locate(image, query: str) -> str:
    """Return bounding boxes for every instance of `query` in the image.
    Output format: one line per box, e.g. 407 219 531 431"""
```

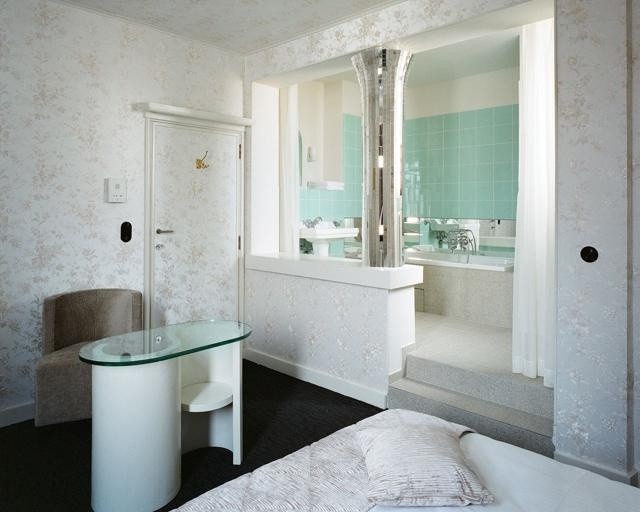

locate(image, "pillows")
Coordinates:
354 425 495 504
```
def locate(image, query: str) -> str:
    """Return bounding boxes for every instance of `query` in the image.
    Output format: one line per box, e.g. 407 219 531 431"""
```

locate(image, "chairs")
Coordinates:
34 289 143 427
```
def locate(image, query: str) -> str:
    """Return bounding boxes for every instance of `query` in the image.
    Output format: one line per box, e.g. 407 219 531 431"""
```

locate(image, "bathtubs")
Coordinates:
404 245 515 272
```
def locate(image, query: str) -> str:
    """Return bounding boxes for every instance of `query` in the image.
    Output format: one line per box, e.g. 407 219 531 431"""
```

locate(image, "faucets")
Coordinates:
409 245 422 253
303 215 323 228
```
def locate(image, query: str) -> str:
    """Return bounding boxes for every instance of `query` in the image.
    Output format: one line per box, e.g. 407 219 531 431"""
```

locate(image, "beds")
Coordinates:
166 406 640 510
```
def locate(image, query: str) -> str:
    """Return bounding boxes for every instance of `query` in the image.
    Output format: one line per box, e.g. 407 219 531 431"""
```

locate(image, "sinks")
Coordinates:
299 227 360 239
